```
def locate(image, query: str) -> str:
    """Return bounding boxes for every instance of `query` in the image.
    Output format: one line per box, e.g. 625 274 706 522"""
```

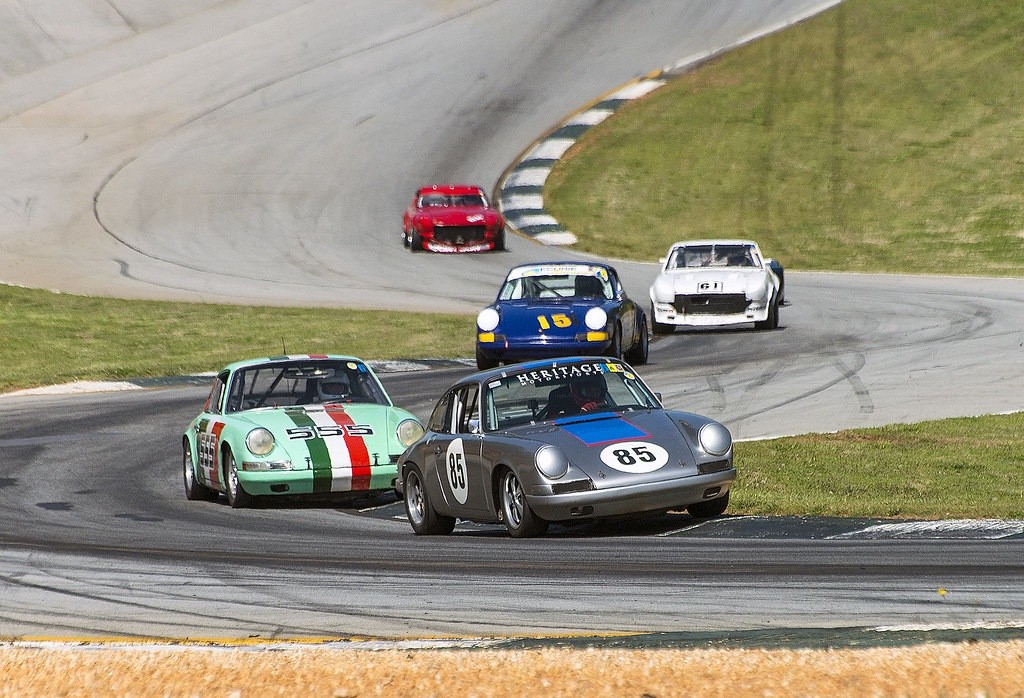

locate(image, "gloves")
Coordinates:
579 401 597 412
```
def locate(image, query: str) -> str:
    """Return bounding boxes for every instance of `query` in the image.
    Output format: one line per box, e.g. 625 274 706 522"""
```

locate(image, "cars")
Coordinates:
395 355 737 538
683 247 787 307
401 183 507 255
182 334 428 511
651 240 782 336
474 261 650 380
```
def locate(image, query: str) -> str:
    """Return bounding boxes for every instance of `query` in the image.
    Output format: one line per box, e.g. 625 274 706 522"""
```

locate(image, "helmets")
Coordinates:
463 196 478 205
568 372 608 399
574 276 596 292
316 370 351 400
727 248 747 266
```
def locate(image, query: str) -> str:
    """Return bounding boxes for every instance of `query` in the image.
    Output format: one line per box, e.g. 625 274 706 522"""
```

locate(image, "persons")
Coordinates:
556 375 607 418
314 370 353 405
